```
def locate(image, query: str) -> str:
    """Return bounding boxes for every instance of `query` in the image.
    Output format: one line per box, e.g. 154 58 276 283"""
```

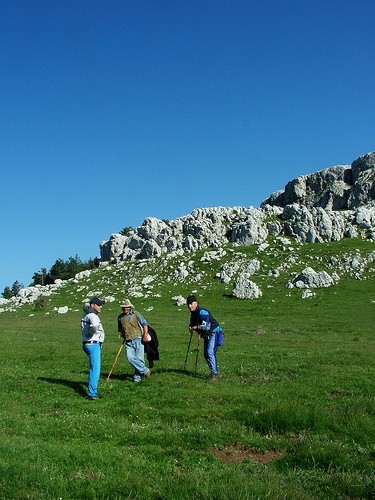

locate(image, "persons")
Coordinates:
81 298 105 400
186 295 220 381
118 299 151 383
142 326 159 368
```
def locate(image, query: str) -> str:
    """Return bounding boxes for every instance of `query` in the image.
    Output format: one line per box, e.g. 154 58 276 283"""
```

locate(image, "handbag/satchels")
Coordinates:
217 332 225 346
141 328 151 342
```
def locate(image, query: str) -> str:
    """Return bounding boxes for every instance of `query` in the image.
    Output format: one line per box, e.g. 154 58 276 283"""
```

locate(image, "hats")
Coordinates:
120 299 133 307
187 295 199 304
90 298 105 306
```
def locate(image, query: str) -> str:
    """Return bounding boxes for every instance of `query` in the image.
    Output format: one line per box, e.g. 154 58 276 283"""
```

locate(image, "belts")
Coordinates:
83 341 102 344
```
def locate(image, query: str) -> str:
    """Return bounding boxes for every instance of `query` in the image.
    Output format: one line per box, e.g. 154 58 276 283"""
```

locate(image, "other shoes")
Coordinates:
209 373 218 382
88 397 97 400
133 380 137 385
146 371 151 379
